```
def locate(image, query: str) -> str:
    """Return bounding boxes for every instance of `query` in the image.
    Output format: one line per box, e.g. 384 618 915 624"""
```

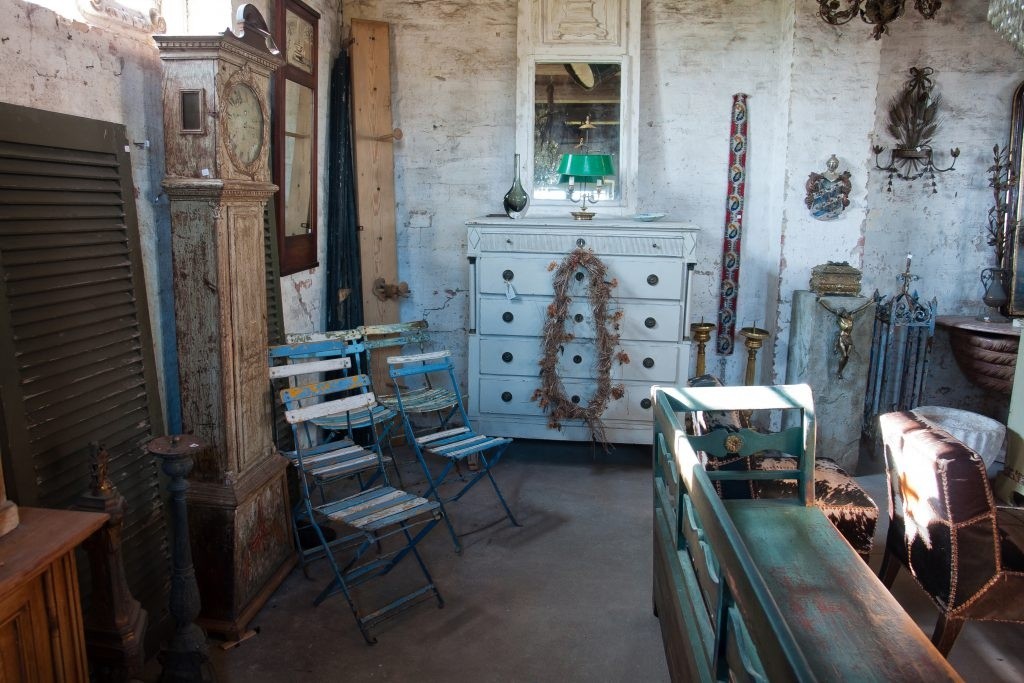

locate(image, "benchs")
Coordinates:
643 384 967 682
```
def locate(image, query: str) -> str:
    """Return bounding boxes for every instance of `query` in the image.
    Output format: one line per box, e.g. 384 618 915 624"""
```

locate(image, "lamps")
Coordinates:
555 152 617 221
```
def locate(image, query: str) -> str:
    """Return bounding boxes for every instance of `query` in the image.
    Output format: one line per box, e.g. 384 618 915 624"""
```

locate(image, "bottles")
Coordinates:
503 154 529 219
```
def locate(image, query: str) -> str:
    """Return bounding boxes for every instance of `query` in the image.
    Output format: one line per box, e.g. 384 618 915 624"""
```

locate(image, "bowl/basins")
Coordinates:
914 406 1006 471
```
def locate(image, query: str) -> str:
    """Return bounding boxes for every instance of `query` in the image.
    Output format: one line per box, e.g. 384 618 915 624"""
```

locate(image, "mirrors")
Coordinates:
532 61 623 204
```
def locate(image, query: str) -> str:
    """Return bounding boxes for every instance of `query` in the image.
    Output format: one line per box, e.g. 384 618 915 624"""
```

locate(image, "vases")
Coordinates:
503 153 530 219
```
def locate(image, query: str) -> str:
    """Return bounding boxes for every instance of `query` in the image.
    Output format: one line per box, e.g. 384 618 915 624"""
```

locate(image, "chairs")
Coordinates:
689 375 879 566
877 411 1024 657
269 319 523 645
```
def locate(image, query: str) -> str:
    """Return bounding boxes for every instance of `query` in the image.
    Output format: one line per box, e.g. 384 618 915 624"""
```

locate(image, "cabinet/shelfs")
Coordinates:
0 507 111 683
464 217 700 444
